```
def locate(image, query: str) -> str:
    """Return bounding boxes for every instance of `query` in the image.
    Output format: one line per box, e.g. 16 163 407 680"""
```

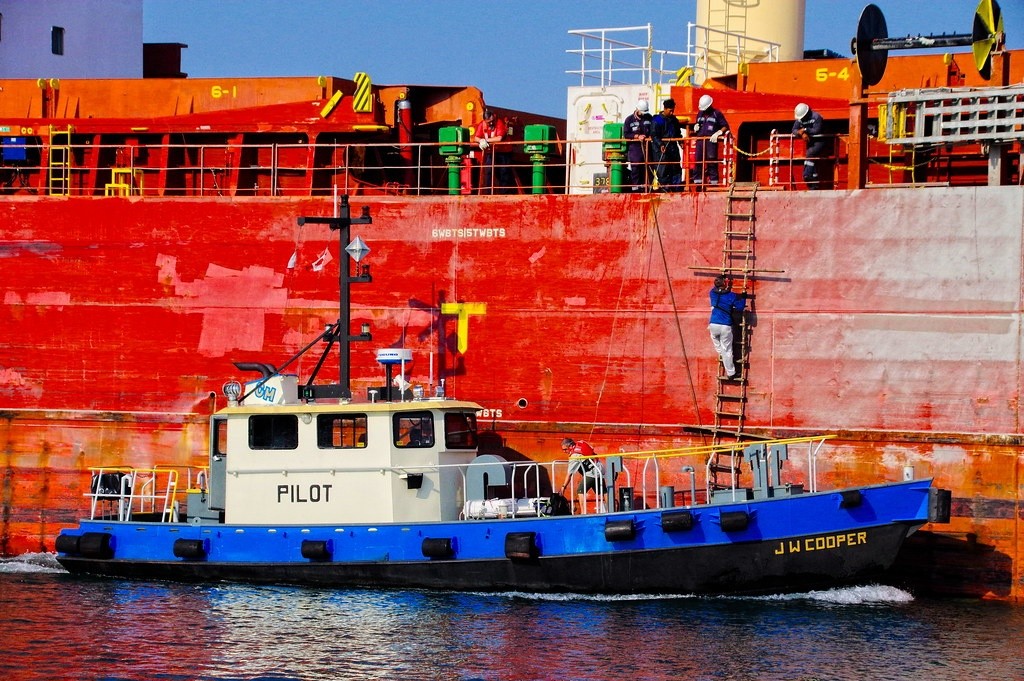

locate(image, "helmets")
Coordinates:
698 95 713 110
793 103 809 119
637 100 649 110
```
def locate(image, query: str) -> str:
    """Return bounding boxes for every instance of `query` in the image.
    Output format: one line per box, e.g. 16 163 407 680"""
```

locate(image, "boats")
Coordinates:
54 195 944 598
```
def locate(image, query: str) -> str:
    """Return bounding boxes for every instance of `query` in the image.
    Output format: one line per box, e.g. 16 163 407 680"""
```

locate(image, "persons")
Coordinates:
649 98 686 191
558 437 610 516
623 100 655 192
473 110 513 194
708 276 749 380
692 93 729 190
792 102 827 190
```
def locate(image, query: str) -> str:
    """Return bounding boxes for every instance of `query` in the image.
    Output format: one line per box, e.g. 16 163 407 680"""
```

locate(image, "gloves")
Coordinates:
741 291 747 298
694 123 700 132
710 130 723 143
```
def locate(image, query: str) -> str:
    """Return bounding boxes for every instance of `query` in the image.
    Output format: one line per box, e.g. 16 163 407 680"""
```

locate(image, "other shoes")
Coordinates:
728 373 741 379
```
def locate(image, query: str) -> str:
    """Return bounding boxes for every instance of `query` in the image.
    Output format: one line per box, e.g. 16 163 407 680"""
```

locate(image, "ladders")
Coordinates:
704 184 760 494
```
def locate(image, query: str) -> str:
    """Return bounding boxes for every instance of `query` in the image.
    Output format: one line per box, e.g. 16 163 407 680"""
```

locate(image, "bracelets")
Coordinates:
562 486 567 489
